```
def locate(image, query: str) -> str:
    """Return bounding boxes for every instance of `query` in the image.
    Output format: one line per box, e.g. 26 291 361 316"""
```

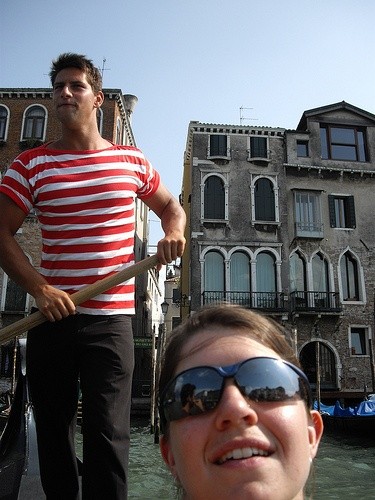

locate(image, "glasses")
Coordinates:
161 356 312 425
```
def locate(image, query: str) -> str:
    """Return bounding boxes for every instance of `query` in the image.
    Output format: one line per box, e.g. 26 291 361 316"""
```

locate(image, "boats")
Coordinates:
309 393 375 436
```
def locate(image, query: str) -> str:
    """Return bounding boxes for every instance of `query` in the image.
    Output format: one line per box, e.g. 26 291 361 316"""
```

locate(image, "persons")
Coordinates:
0 54 186 499
275 385 290 401
173 383 205 420
155 302 323 499
